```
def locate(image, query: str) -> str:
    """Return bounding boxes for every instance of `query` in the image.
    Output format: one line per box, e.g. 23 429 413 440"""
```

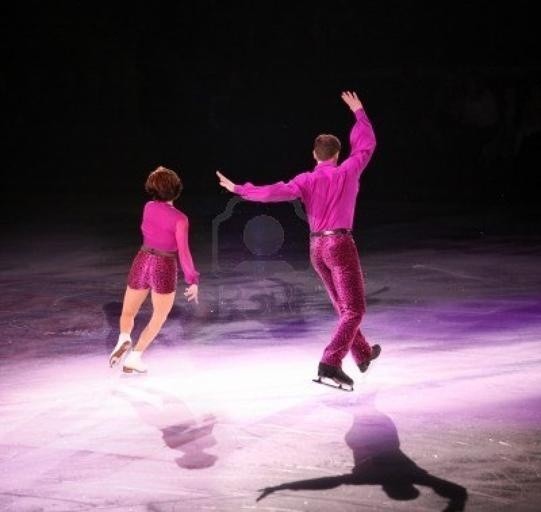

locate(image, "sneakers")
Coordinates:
318 363 353 385
122 350 146 373
358 345 379 373
109 336 131 369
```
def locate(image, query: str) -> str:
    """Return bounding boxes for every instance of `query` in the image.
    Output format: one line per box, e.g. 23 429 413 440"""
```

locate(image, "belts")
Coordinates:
309 228 353 237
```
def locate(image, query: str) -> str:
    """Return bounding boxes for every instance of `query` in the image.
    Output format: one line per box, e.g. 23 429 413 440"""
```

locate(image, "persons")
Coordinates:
108 168 199 373
215 90 380 388
